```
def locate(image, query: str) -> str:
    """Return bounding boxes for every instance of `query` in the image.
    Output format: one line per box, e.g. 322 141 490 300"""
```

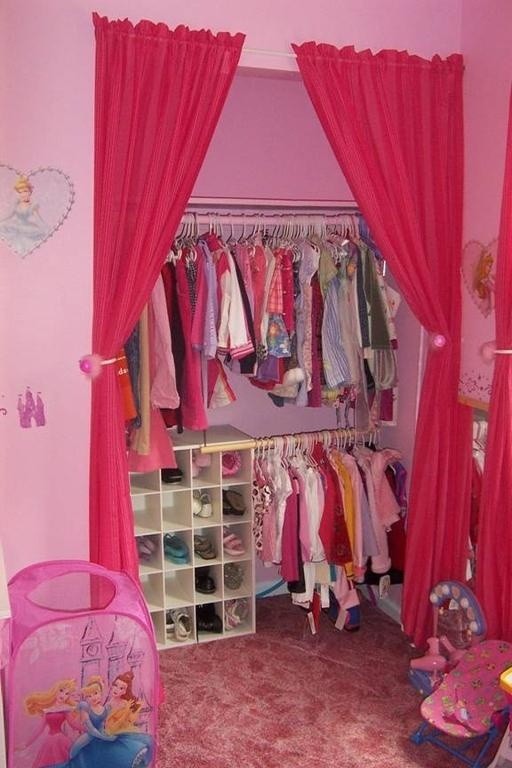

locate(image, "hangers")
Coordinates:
165 203 371 249
247 420 386 470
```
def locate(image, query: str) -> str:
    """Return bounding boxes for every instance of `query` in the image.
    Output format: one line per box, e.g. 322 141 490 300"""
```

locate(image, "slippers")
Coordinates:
136 533 190 563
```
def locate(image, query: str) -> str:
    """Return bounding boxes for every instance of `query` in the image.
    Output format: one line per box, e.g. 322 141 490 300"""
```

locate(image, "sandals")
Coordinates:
166 607 192 641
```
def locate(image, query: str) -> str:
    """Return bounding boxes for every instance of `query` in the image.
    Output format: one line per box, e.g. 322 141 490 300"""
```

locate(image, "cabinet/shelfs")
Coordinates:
120 426 261 653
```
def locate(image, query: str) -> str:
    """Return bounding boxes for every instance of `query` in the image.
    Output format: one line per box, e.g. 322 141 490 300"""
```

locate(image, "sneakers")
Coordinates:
161 468 182 484
192 449 248 632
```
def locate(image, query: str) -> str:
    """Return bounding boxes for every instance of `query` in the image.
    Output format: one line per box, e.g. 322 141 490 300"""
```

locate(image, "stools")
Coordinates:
406 637 512 767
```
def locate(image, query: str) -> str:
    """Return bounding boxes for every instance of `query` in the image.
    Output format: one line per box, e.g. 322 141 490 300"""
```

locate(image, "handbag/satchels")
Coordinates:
0 560 159 766
